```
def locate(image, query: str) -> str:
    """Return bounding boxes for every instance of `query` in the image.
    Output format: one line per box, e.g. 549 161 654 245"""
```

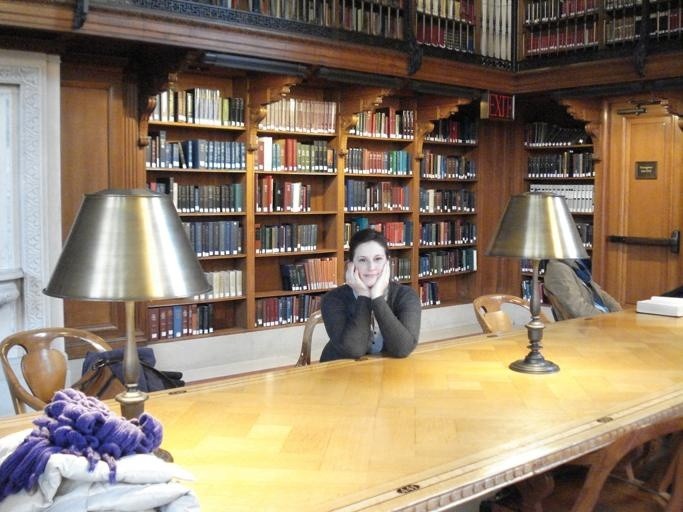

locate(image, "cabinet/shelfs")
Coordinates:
133 69 484 343
520 98 606 307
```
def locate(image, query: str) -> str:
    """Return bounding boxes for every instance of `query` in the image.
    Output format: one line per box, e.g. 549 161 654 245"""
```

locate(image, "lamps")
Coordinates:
486 189 588 376
42 187 215 466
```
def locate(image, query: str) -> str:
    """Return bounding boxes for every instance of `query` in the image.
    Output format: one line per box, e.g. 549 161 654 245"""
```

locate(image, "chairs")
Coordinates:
473 294 551 338
0 323 128 414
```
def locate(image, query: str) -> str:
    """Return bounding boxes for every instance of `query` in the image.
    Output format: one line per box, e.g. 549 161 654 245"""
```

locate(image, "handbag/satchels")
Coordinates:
68 357 130 403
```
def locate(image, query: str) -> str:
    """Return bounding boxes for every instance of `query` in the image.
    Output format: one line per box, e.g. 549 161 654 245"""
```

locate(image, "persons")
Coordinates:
318 228 420 361
543 259 622 322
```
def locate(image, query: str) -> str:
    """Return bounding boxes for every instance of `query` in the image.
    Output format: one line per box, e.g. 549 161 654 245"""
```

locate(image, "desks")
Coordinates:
0 307 681 511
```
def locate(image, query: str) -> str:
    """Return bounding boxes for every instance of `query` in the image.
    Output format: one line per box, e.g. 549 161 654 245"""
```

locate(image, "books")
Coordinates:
634 296 682 318
521 118 594 305
